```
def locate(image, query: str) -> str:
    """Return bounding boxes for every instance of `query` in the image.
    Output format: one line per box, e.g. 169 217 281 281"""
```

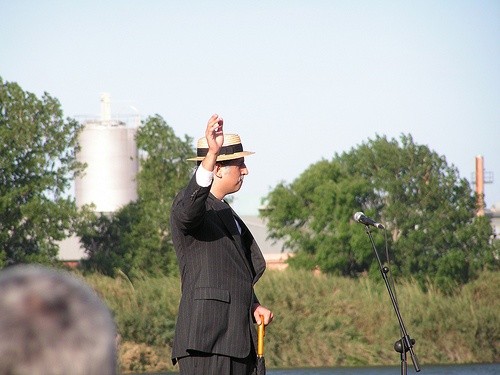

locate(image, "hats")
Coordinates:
187 133 255 161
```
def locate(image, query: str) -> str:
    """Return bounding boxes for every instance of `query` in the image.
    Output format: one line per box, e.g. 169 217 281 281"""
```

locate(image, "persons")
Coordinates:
0 264 122 374
170 112 276 375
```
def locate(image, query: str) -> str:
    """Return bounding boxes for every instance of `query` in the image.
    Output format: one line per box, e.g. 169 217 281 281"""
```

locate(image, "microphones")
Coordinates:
353 211 384 229
